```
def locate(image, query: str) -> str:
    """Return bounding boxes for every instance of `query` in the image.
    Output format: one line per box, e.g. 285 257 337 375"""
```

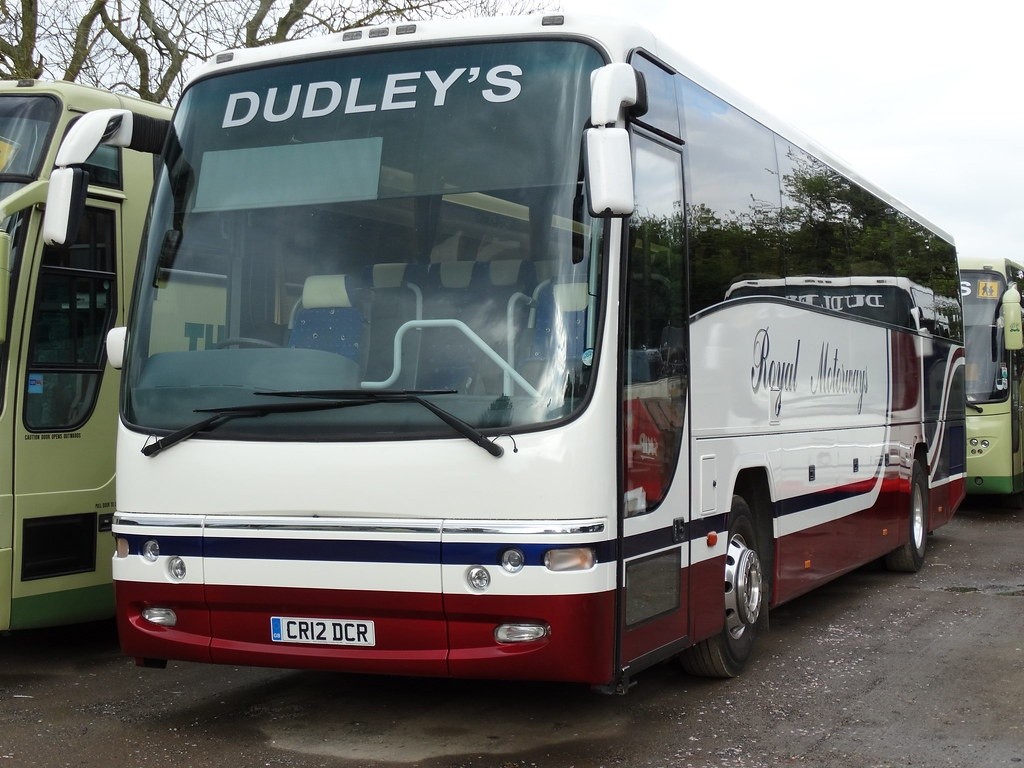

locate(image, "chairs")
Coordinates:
285 259 904 399
965 363 982 391
24 313 79 430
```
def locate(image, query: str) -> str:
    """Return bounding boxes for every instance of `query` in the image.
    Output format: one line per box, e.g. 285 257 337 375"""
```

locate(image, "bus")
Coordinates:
725 275 962 473
0 82 680 637
36 11 966 691
958 258 1024 494
